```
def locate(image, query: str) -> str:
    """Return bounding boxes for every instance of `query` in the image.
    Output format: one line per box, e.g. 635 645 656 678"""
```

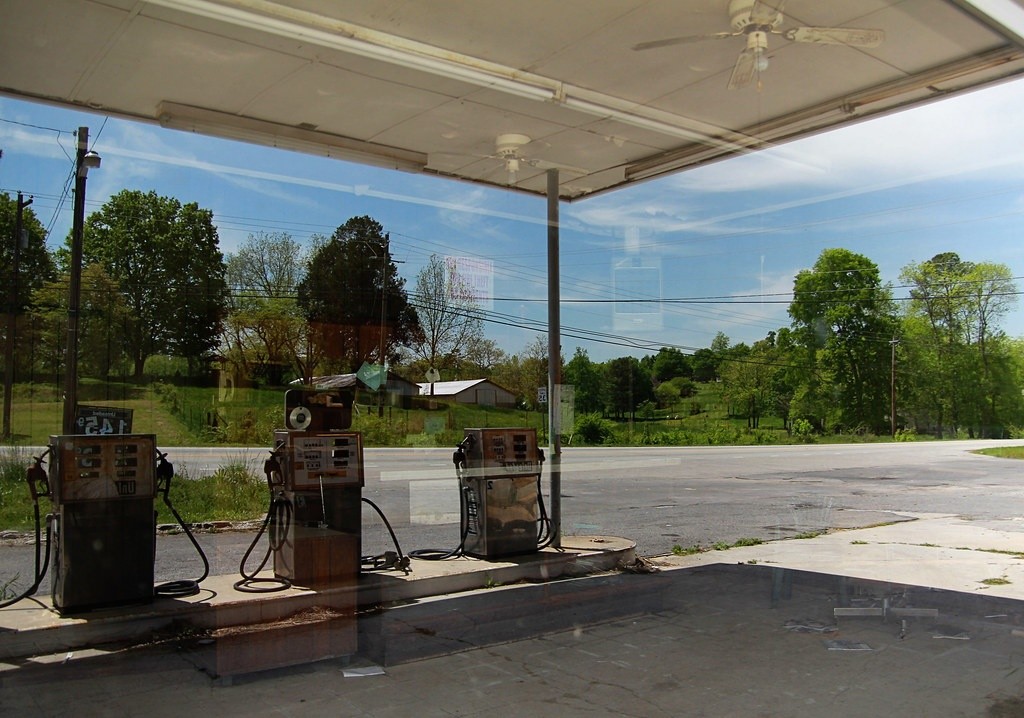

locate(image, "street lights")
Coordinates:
64 152 102 434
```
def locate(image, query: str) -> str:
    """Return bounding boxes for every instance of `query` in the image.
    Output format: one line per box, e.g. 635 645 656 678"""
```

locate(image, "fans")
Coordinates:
431 135 588 188
637 0 885 94
